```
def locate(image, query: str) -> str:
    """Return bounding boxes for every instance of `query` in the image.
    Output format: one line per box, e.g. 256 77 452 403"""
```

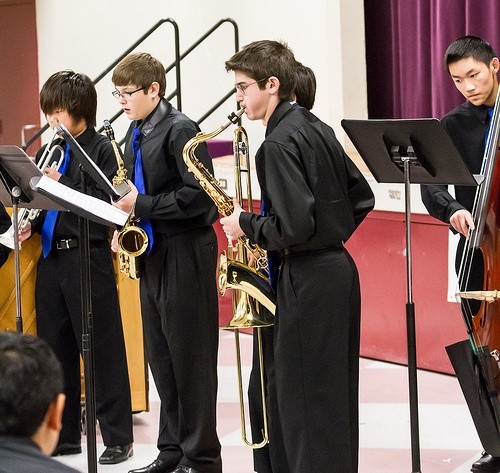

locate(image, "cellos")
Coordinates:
451 81 500 458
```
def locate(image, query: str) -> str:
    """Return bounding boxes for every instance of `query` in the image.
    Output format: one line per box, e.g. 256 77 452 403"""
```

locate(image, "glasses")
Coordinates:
111 87 144 99
234 78 266 94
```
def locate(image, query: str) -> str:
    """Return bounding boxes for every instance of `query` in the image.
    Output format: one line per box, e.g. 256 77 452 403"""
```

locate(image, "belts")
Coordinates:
283 241 344 255
52 238 80 250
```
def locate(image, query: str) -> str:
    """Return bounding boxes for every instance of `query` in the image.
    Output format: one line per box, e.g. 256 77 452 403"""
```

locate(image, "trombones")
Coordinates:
231 125 270 449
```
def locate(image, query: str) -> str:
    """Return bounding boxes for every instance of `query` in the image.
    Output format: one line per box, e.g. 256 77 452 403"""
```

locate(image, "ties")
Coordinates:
41 143 71 259
131 128 153 256
260 193 280 295
485 108 494 149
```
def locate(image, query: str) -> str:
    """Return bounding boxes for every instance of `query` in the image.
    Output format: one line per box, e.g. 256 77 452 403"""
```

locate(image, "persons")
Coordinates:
0 330 81 472
220 40 375 473
420 35 500 473
18 71 134 465
111 52 222 473
0 201 31 270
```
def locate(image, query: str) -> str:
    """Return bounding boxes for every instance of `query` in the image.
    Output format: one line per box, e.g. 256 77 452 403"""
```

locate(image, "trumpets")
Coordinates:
0 129 64 250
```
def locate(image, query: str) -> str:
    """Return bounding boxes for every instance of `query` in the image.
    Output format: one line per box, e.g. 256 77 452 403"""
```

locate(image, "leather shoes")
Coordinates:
470 450 500 473
128 458 176 473
98 442 134 464
171 464 199 473
50 447 81 457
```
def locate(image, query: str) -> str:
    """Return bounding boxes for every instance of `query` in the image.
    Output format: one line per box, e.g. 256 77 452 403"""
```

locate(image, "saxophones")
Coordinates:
103 119 149 280
182 107 278 315
